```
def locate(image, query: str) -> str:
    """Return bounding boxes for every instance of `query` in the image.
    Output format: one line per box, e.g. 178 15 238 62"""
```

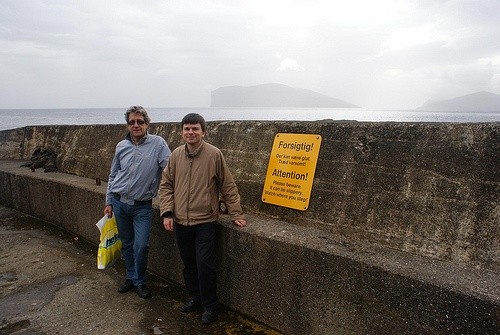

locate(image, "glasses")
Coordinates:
128 119 146 125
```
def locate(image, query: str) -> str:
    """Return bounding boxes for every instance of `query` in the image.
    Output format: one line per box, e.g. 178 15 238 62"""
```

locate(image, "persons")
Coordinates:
158 113 247 324
103 105 172 298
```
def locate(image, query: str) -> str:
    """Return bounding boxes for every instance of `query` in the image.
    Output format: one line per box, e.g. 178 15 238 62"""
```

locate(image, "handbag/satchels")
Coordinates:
96 211 123 270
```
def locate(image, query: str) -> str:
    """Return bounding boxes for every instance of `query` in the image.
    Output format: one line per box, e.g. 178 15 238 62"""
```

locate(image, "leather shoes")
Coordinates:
180 298 221 325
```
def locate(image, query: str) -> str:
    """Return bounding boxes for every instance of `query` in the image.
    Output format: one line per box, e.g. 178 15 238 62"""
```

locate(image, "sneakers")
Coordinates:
119 278 152 299
